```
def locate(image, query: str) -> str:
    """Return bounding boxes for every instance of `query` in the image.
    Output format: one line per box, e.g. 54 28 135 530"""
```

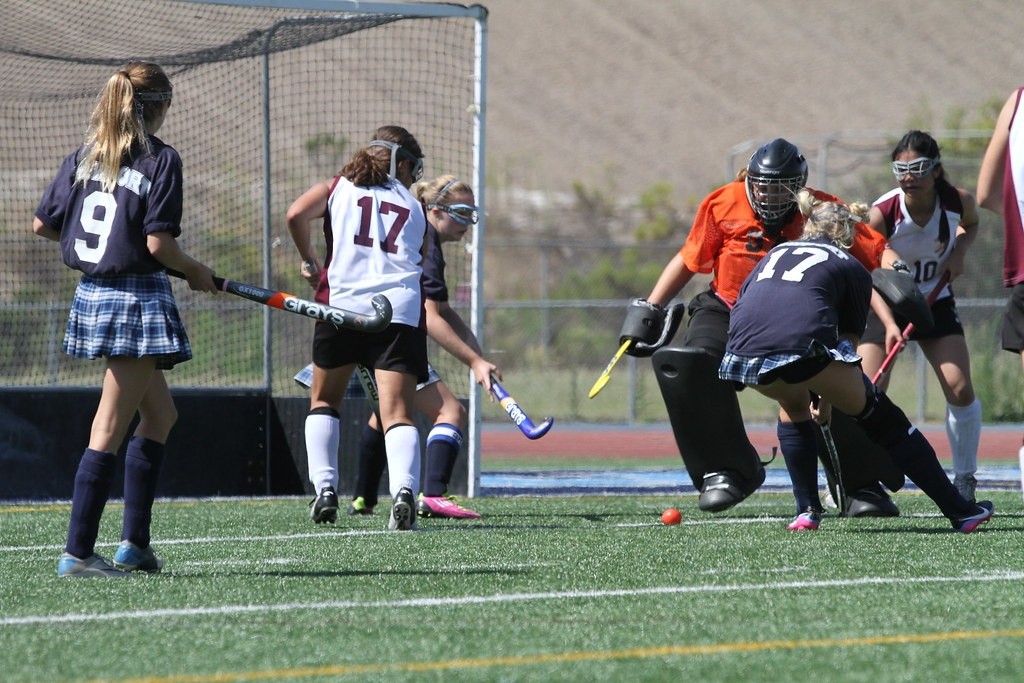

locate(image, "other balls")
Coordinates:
662 509 682 525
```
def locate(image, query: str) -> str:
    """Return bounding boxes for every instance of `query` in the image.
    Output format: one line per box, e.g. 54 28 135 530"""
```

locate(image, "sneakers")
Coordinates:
347 495 373 515
58 553 134 578
112 539 163 574
416 491 480 519
950 500 994 532
309 485 340 524
388 487 417 530
786 506 829 531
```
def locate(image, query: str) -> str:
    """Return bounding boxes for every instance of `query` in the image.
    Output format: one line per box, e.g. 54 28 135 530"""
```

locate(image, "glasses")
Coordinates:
892 154 939 182
426 203 479 225
366 140 423 183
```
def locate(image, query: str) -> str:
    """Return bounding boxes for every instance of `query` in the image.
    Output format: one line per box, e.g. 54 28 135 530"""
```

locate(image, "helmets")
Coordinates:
748 137 809 187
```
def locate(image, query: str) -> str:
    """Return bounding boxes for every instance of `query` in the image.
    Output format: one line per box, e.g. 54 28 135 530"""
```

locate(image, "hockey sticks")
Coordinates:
804 391 851 515
486 378 554 440
587 339 635 399
869 270 953 388
162 266 393 331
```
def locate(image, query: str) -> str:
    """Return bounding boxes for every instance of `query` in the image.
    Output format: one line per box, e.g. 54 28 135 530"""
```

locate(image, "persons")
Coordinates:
286 126 428 532
718 183 994 534
854 129 983 505
618 136 936 517
32 59 217 577
293 175 504 519
976 87 1024 503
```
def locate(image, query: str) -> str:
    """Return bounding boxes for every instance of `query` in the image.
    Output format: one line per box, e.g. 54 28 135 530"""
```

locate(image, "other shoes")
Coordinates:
953 472 977 503
844 483 900 517
697 447 777 512
